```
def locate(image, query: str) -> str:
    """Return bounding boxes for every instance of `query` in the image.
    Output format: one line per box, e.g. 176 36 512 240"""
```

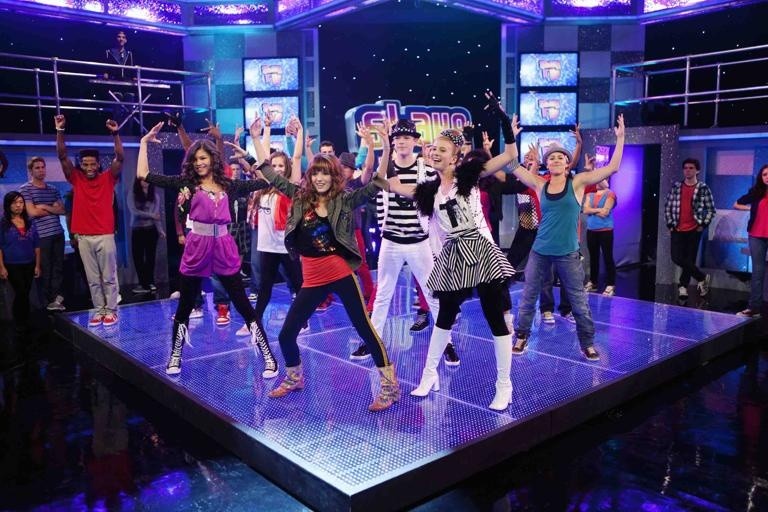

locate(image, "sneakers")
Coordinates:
677 274 760 317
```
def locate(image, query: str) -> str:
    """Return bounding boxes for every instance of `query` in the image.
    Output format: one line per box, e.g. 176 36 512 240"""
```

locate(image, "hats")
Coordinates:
389 118 420 137
541 142 572 166
338 152 356 170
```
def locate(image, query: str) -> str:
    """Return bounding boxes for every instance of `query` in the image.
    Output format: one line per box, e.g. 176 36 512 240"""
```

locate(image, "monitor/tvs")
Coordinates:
517 130 577 168
538 168 578 175
515 90 579 130
242 56 301 94
243 133 296 162
242 95 301 132
516 48 582 91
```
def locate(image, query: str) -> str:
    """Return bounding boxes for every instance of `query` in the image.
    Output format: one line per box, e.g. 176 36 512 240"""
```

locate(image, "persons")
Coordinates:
732 164 768 318
83 380 145 510
102 29 135 135
512 113 624 360
662 158 716 297
54 114 126 327
1 157 166 331
136 90 518 414
